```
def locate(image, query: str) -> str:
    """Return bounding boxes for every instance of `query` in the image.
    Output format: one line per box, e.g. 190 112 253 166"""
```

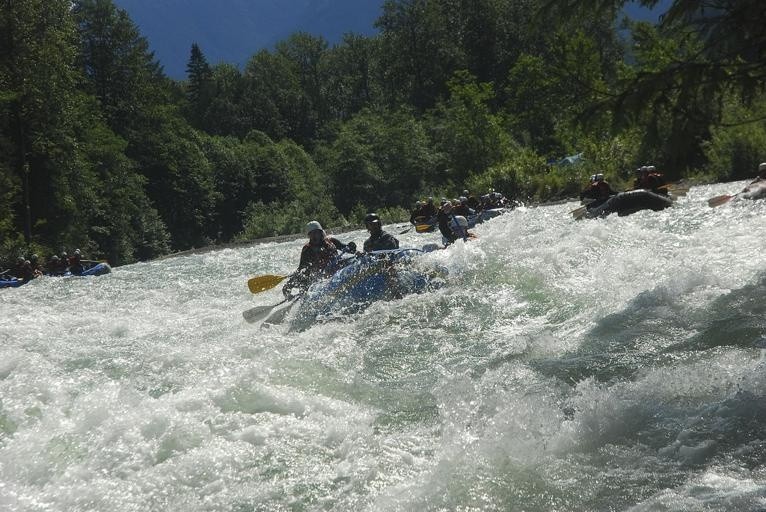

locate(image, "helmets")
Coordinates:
306 220 323 234
416 196 433 206
482 193 502 199
635 166 656 175
450 215 468 228
759 163 766 171
589 174 604 182
52 249 79 259
364 213 381 224
17 254 39 265
440 190 469 209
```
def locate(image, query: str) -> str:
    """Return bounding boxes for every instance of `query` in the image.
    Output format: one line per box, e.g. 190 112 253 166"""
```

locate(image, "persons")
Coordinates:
438 201 477 247
362 213 399 253
409 187 503 225
742 162 766 192
15 248 91 283
281 220 357 300
579 165 668 211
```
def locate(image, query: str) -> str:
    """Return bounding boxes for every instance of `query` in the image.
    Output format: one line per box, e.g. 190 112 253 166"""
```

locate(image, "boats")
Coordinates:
585 189 673 219
414 206 509 233
0 261 112 288
291 247 444 332
732 183 766 203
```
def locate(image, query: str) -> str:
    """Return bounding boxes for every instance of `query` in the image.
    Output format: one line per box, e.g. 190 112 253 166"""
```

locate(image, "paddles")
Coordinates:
671 188 688 193
243 293 301 323
248 256 355 293
417 225 433 230
399 226 416 234
708 194 738 207
657 185 676 190
260 297 301 331
572 208 587 217
